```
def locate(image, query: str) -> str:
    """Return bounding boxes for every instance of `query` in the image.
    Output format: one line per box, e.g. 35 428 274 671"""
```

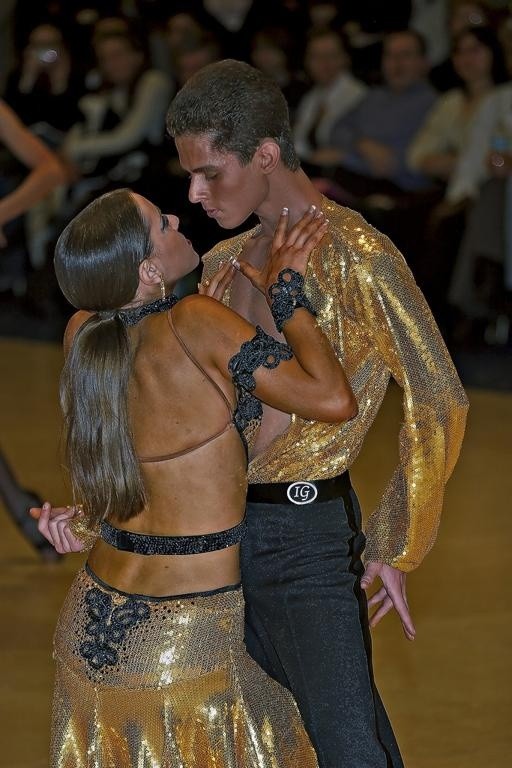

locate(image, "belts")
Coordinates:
243 468 351 507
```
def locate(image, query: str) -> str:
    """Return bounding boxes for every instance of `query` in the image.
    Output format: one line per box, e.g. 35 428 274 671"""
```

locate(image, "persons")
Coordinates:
44 189 356 766
1 97 69 564
1 4 512 396
26 58 471 768
